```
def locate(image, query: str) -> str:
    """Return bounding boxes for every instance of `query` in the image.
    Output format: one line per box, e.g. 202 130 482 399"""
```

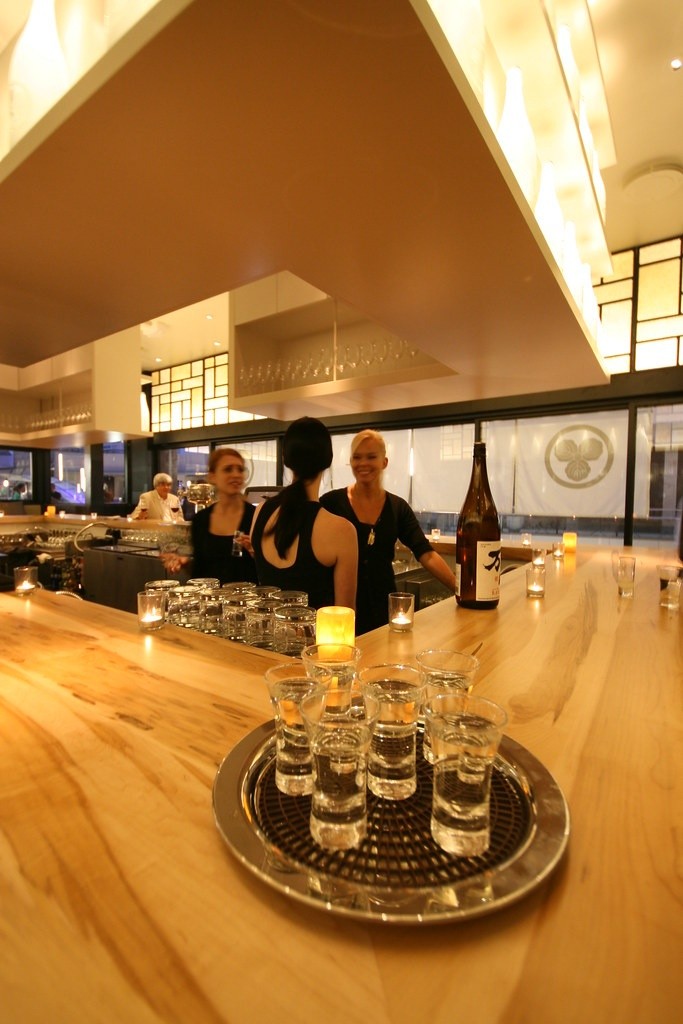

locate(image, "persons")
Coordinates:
251 418 357 615
163 448 267 588
49 483 62 503
131 473 185 521
13 483 26 499
103 483 113 502
318 428 454 636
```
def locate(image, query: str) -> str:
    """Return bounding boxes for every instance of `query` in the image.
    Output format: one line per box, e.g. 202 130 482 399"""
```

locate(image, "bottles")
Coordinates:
455 442 504 608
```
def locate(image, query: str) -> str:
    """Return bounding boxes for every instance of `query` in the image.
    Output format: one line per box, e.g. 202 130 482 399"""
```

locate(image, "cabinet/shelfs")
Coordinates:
228 273 457 422
0 323 153 449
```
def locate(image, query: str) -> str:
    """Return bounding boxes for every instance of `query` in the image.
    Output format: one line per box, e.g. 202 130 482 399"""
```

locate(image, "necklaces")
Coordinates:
360 511 383 547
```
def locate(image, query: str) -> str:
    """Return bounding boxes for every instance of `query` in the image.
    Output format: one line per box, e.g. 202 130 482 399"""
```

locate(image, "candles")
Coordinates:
563 532 576 552
316 607 356 661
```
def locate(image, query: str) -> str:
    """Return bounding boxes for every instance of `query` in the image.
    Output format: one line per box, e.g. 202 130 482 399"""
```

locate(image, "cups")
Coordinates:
526 567 545 598
657 565 683 610
416 648 481 764
552 541 565 560
121 529 159 544
422 694 507 858
353 664 431 800
301 643 362 719
14 566 39 597
299 689 384 849
0 505 133 523
136 578 318 656
432 528 440 541
264 662 341 794
522 532 532 547
532 547 546 573
620 558 636 596
389 592 414 633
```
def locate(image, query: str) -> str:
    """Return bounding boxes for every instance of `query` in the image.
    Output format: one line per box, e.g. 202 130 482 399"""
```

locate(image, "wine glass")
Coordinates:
170 498 179 523
140 498 148 519
